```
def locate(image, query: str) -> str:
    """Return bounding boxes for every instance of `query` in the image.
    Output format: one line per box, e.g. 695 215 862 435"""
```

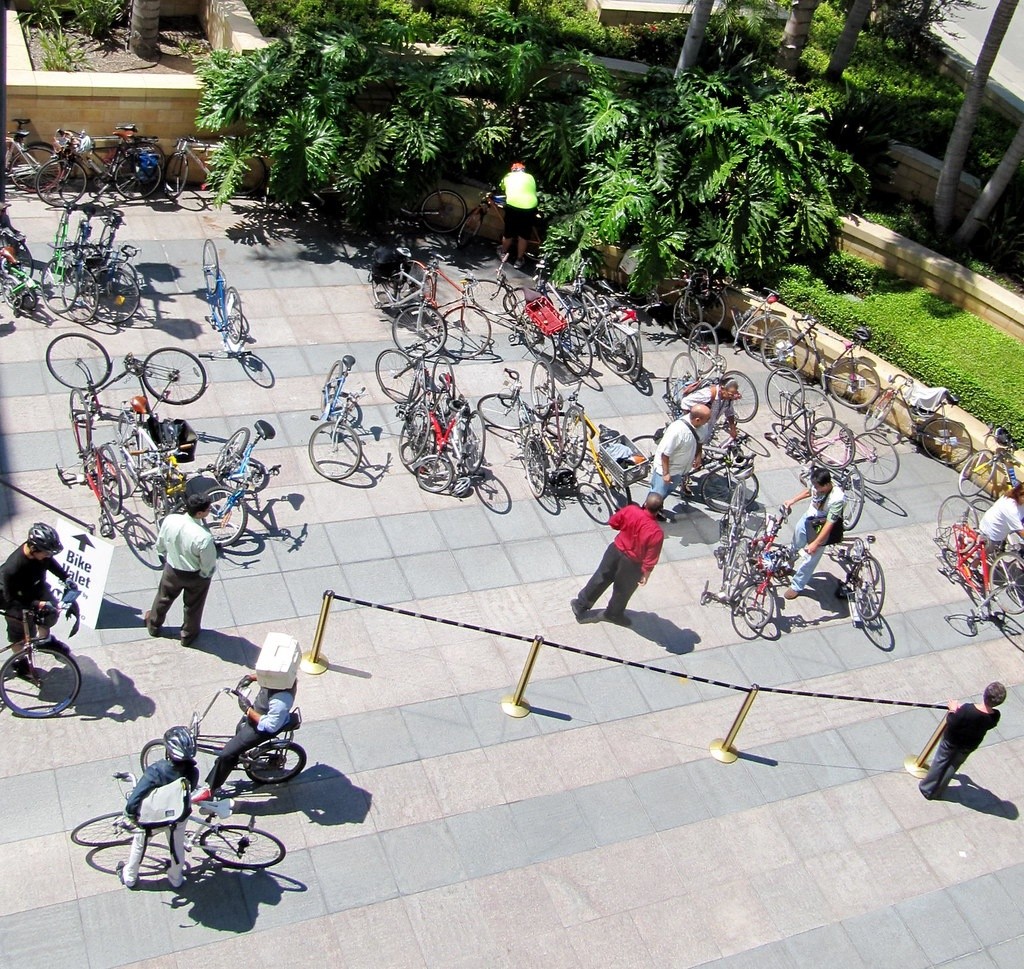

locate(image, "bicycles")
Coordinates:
139 685 307 786
0 588 83 719
70 772 287 871
0 118 1023 631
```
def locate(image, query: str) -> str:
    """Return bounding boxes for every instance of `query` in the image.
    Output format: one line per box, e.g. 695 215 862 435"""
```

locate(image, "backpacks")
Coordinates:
682 376 719 407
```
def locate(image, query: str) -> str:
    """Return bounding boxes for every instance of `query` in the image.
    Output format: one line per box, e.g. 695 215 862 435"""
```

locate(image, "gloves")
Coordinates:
34 600 56 613
239 675 252 687
65 577 78 591
238 695 252 715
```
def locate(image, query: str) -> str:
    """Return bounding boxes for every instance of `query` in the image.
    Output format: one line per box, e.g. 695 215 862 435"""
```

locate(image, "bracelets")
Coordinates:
247 707 252 717
949 710 956 712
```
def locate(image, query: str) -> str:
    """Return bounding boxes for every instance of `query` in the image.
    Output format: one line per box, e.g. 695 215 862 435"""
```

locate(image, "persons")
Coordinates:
774 466 845 599
116 726 200 891
968 482 1024 583
645 403 712 521
145 492 216 647
488 163 537 269
919 681 1007 801
190 633 297 819
569 492 664 628
0 522 79 682
680 376 739 499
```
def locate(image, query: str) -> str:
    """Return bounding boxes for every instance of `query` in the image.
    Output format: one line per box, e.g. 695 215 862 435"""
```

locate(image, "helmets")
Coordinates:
850 326 870 341
764 551 781 571
994 426 1012 446
29 523 63 555
511 163 525 171
734 465 754 481
448 477 471 497
163 726 196 762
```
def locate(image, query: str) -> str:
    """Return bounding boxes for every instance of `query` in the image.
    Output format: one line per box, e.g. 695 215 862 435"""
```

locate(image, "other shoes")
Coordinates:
774 567 793 577
784 587 801 599
571 599 584 617
14 611 220 892
604 610 631 626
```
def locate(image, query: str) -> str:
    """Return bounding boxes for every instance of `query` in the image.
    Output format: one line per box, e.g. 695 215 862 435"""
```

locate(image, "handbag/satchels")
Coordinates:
805 516 843 546
135 777 192 830
370 246 411 287
138 152 158 178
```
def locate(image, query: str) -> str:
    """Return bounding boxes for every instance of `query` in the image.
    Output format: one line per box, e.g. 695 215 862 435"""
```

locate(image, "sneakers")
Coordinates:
970 567 984 582
514 257 525 268
496 248 508 262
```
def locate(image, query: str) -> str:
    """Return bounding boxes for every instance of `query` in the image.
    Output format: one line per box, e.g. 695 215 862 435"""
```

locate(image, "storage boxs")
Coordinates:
597 435 650 488
255 633 303 690
